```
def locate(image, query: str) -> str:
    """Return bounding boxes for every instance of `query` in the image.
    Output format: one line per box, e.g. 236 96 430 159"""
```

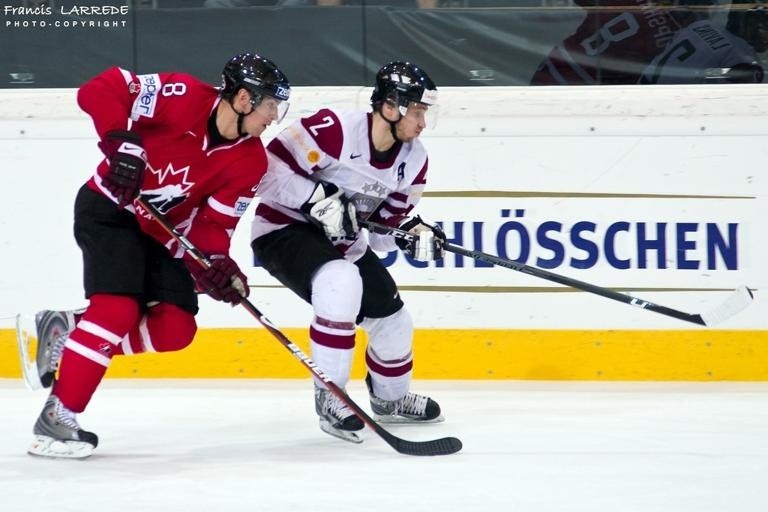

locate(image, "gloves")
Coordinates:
94 134 147 210
195 254 250 308
301 183 360 245
395 213 446 260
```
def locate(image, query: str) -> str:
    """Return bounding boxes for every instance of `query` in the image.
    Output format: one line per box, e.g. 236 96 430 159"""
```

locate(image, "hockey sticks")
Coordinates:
357 219 753 327
134 193 462 456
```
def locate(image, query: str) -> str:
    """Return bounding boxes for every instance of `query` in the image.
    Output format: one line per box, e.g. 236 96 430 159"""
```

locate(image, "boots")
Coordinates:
365 371 441 420
314 385 364 432
35 307 70 389
33 395 98 447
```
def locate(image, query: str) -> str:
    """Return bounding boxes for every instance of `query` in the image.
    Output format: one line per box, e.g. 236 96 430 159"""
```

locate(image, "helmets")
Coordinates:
221 52 292 107
370 61 438 110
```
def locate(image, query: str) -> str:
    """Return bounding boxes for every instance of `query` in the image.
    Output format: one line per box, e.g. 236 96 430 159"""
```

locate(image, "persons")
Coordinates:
527 0 719 85
31 50 289 450
249 60 447 431
634 0 767 84
